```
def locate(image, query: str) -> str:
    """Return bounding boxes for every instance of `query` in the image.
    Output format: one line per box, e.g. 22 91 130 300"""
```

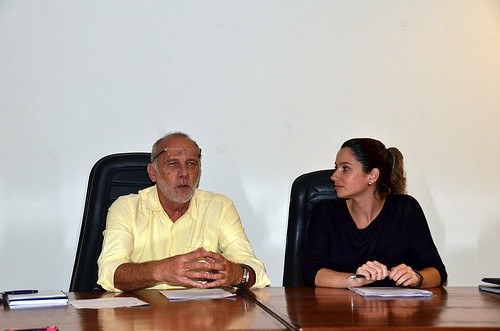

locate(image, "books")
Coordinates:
478 278 500 293
6 290 69 305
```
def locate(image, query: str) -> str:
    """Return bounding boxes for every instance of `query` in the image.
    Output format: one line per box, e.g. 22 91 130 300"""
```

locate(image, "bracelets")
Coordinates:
408 271 423 289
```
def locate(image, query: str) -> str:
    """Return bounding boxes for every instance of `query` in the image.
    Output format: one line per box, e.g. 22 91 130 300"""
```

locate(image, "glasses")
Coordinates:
151 146 202 163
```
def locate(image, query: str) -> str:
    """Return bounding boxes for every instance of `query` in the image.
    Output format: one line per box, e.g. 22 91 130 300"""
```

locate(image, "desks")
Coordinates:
0 288 289 331
242 286 500 331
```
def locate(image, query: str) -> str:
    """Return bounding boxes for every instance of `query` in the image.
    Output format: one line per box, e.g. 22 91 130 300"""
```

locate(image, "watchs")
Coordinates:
229 266 249 290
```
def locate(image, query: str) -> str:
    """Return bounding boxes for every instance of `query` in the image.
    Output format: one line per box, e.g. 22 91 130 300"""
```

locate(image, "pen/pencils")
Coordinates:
349 271 390 278
3 326 60 331
3 289 39 295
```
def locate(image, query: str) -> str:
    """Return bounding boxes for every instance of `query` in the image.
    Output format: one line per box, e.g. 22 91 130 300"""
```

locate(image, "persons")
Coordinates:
301 138 447 289
97 132 272 293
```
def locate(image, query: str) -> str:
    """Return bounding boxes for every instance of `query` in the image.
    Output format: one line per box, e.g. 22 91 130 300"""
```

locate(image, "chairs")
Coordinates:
283 170 338 284
69 153 155 291
